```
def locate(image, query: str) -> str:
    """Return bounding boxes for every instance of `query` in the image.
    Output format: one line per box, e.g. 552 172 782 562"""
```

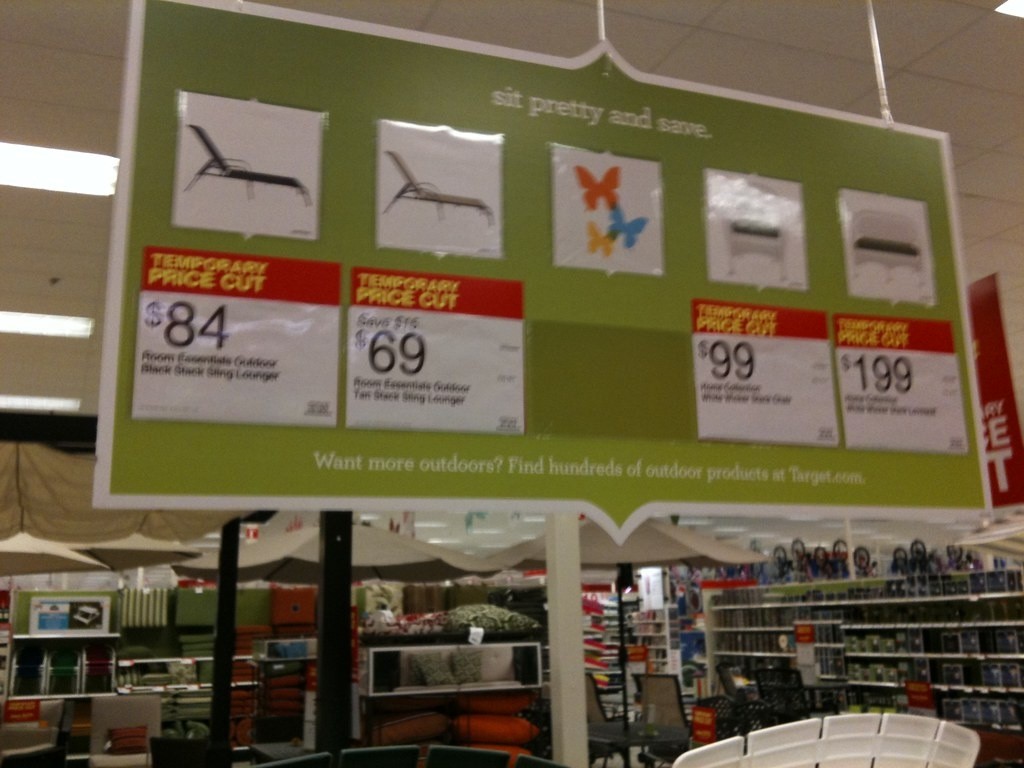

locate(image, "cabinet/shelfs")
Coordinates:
0 585 318 768
357 643 543 698
580 539 1024 768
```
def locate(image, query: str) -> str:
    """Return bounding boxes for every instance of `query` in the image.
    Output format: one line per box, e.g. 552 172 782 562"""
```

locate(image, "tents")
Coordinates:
11 411 358 768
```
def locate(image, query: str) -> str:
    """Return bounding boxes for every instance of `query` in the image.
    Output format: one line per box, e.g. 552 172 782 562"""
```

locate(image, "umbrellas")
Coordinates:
0 499 769 767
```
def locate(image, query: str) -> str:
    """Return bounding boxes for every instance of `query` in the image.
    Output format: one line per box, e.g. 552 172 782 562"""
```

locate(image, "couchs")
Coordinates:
0 697 74 768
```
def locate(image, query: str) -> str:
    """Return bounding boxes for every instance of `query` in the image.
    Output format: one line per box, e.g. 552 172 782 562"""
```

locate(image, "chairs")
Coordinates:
87 694 163 768
584 672 631 768
630 672 696 768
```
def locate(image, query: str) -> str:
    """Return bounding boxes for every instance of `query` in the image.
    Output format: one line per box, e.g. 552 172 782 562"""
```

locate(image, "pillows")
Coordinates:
448 650 482 684
355 585 547 640
365 691 541 765
109 725 150 755
408 654 426 687
416 652 455 687
